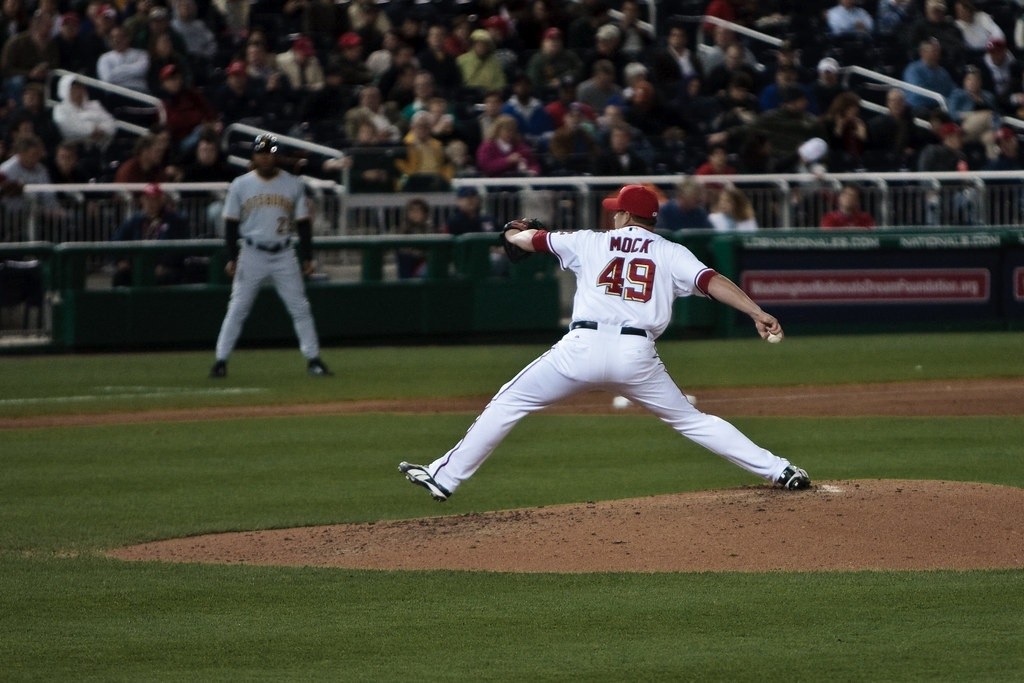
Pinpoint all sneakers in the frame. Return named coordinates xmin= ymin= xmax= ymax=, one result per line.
xmin=398 ymin=460 xmax=451 ymax=504
xmin=776 ymin=463 xmax=811 ymax=491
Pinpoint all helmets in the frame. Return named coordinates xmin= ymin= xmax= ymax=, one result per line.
xmin=250 ymin=132 xmax=279 ymax=154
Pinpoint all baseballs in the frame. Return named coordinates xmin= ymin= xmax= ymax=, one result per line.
xmin=766 ymin=331 xmax=783 ymax=344
xmin=612 ymin=396 xmax=631 ymax=410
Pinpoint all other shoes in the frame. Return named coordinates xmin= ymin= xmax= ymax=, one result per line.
xmin=307 ymin=359 xmax=327 ymax=375
xmin=210 ymin=360 xmax=226 ymax=378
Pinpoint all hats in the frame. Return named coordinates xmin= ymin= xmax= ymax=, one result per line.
xmin=937 ymin=122 xmax=967 ymax=137
xmin=293 ymin=35 xmax=318 ymax=57
xmin=60 ymin=15 xmax=81 ymax=29
xmin=471 ymin=30 xmax=491 ymax=44
xmin=335 ymin=31 xmax=365 ymax=48
xmin=995 ymin=128 xmax=1012 ymax=145
xmin=486 ymin=16 xmax=506 ymax=30
xmin=227 ymin=61 xmax=247 ymax=77
xmin=545 ymin=26 xmax=564 ymax=41
xmin=817 ymin=56 xmax=841 ymax=73
xmin=983 ymin=39 xmax=1007 ymax=54
xmin=797 ymin=136 xmax=829 ymax=163
xmin=601 ymin=184 xmax=659 ymax=220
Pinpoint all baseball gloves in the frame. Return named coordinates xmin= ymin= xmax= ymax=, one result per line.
xmin=501 ymin=217 xmax=548 ymax=263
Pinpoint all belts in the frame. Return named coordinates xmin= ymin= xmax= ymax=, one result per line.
xmin=245 ymin=237 xmax=290 ymax=254
xmin=569 ymin=319 xmax=648 ymax=339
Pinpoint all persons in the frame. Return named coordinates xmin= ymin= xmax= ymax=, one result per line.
xmin=0 ymin=0 xmax=1024 ymax=379
xmin=398 ymin=185 xmax=811 ymax=502
